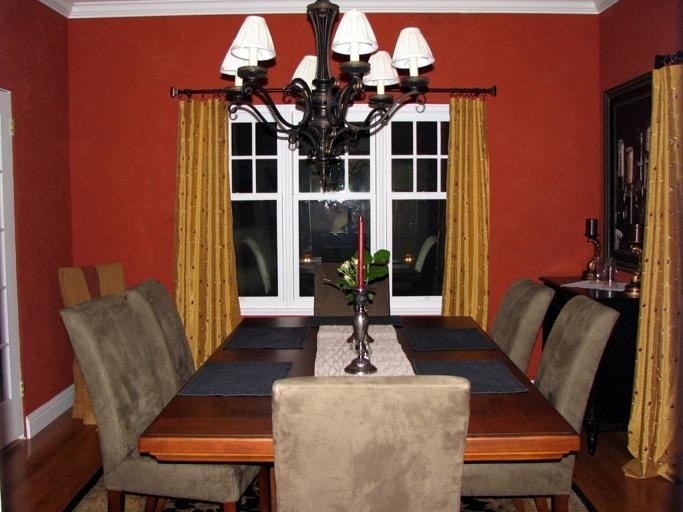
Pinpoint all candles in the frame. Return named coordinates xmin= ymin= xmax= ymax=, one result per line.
xmin=629 ymin=223 xmax=642 ymax=242
xmin=358 ymin=217 xmax=366 ymax=292
xmin=586 ymin=217 xmax=597 ymax=240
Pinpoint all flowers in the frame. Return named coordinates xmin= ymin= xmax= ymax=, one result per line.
xmin=333 ymin=249 xmax=389 ymax=303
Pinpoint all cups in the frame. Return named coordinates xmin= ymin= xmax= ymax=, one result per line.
xmin=605 ymin=267 xmax=615 ymax=288
xmin=596 ymin=257 xmax=604 ymax=284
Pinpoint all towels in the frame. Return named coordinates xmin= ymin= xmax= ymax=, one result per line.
xmin=309 ymin=315 xmax=404 ymax=327
xmin=222 ymin=325 xmax=309 ymax=349
xmin=404 ymin=326 xmax=497 ymax=351
xmin=411 ymin=358 xmax=529 ymax=395
xmin=177 ymin=360 xmax=294 ymax=397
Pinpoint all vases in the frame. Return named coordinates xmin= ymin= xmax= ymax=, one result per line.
xmin=348 ymin=289 xmax=374 ymax=344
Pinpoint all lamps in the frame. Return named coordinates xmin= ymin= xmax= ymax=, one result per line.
xmin=220 ymin=0 xmax=436 ymax=193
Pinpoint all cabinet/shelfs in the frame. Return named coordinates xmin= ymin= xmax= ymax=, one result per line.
xmin=540 ymin=274 xmax=642 ymax=453
xmin=603 ymin=70 xmax=653 ymax=279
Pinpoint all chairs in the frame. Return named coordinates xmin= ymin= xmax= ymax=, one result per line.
xmin=60 ymin=287 xmax=269 ymax=511
xmin=273 ymin=376 xmax=470 ymax=512
xmin=123 ymin=277 xmax=197 ymax=411
xmin=459 ymin=294 xmax=619 ymax=512
xmin=488 ymin=279 xmax=555 ymax=379
xmin=241 ymin=232 xmax=278 ymax=298
xmin=390 ymin=234 xmax=439 ymax=290
